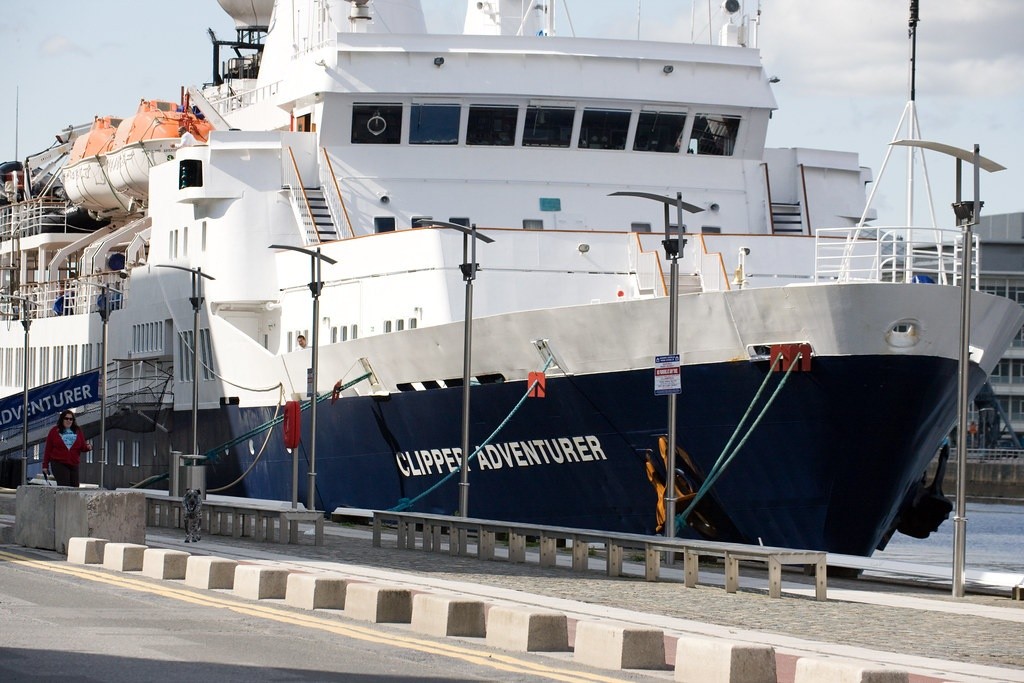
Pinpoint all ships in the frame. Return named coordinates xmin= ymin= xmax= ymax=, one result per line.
xmin=0 ymin=0 xmax=1024 ymax=583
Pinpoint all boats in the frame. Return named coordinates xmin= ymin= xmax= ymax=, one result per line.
xmin=896 ymin=211 xmax=1024 ymax=504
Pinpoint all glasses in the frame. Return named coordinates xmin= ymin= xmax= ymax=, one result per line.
xmin=64 ymin=416 xmax=74 ymax=421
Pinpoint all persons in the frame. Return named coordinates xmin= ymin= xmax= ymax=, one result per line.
xmin=293 ymin=334 xmax=312 ymax=352
xmin=43 ymin=409 xmax=93 ymax=488
